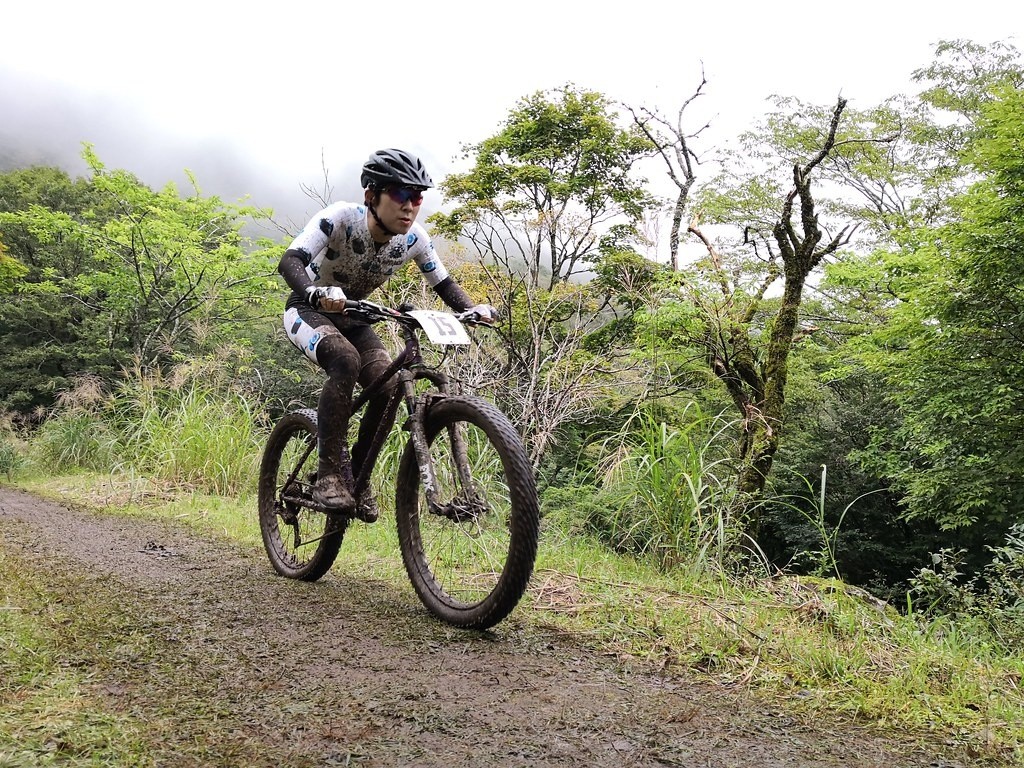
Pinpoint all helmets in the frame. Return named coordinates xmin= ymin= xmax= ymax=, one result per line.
xmin=361 ymin=149 xmax=432 ymax=187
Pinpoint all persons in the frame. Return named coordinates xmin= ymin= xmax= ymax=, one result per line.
xmin=277 ymin=147 xmax=499 ymax=524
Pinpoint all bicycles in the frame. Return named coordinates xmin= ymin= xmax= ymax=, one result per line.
xmin=258 ymin=299 xmax=540 ymax=632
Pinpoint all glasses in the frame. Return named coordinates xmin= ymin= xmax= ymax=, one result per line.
xmin=377 ymin=185 xmax=423 ymax=206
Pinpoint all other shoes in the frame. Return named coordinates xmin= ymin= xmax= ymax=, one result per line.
xmin=313 ymin=474 xmax=353 ymax=510
xmin=347 ymin=477 xmax=379 ymax=522
